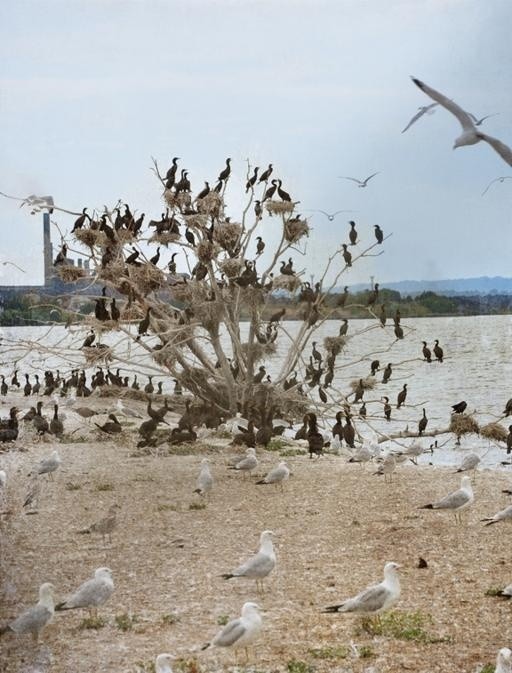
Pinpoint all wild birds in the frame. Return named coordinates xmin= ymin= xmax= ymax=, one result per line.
xmin=22 ymin=471 xmax=42 ymax=510
xmin=400 ymin=74 xmax=512 ymax=167
xmin=0 ymin=582 xmax=60 ymax=640
xmin=192 ymin=458 xmax=216 ymax=506
xmin=415 ymin=475 xmax=476 ymax=524
xmin=319 ymin=562 xmax=405 ymax=627
xmin=215 ymin=530 xmax=283 ymax=594
xmin=55 ymin=567 xmax=118 ymax=619
xmin=479 ymin=505 xmax=512 ymax=527
xmin=1 ymin=157 xmax=512 ymax=492
xmin=494 ymin=583 xmax=512 ymax=601
xmin=495 ymin=646 xmax=512 ymax=673
xmin=155 ymin=654 xmax=183 ymax=673
xmin=200 ymin=602 xmax=268 ymax=666
xmin=73 ymin=502 xmax=122 ymax=546
